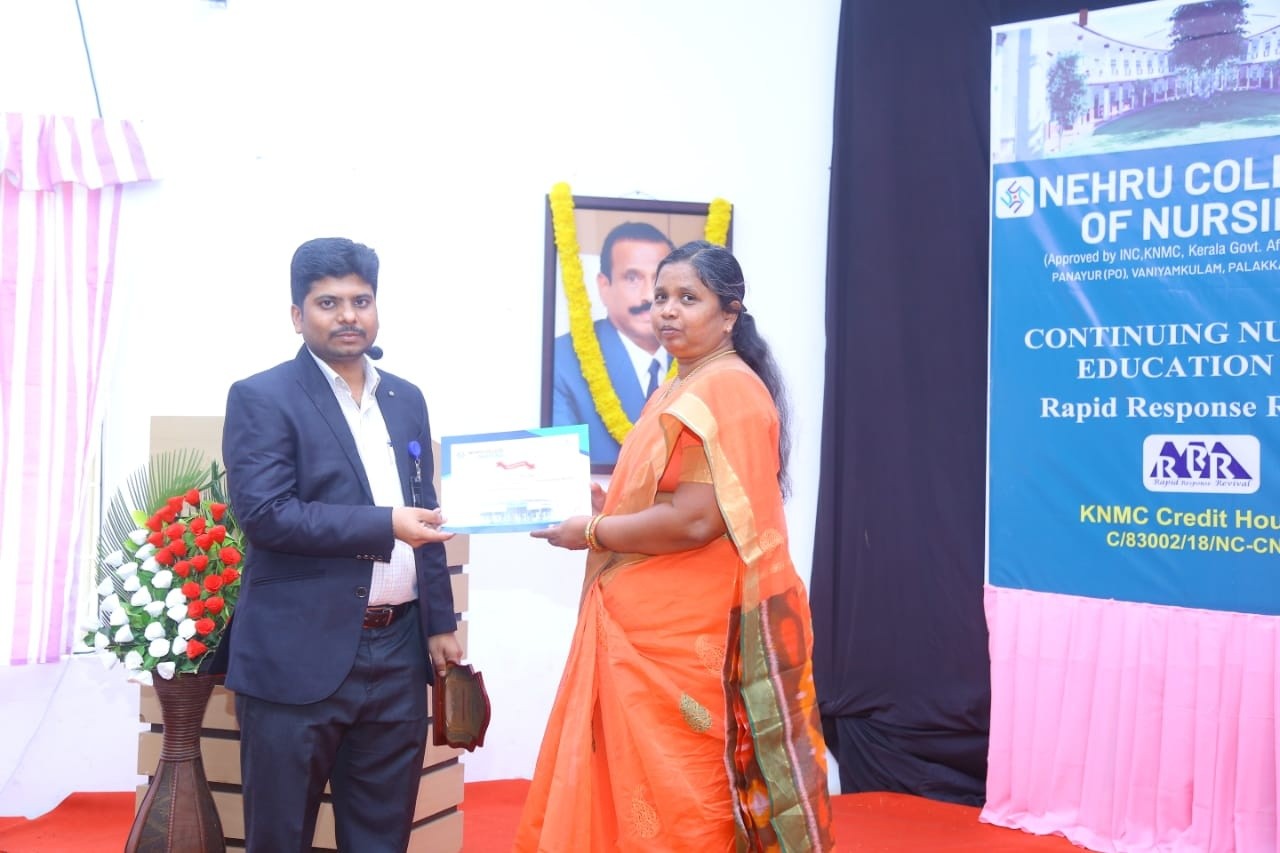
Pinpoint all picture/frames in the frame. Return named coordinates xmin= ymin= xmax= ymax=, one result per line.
xmin=540 ymin=196 xmax=736 ymax=476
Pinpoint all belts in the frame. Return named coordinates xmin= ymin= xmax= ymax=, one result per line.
xmin=362 ymin=602 xmax=412 ymax=629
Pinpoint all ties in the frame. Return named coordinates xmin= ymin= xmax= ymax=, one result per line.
xmin=648 ymin=358 xmax=660 ymax=398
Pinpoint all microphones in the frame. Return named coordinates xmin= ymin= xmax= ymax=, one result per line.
xmin=366 ymin=346 xmax=383 ymax=360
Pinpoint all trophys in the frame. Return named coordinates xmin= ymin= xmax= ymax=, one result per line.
xmin=434 ymin=658 xmax=491 ymax=753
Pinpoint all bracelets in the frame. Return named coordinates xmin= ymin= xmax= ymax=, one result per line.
xmin=584 ymin=512 xmax=613 ymax=553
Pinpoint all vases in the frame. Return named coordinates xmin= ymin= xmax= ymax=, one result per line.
xmin=124 ymin=664 xmax=226 ymax=853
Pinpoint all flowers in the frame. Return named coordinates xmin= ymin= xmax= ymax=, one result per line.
xmin=80 ymin=445 xmax=248 ymax=680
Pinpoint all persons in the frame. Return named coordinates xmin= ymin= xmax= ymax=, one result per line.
xmin=529 ymin=239 xmax=830 ymax=853
xmin=220 ymin=237 xmax=462 ymax=853
xmin=551 ymin=221 xmax=680 ymax=465
